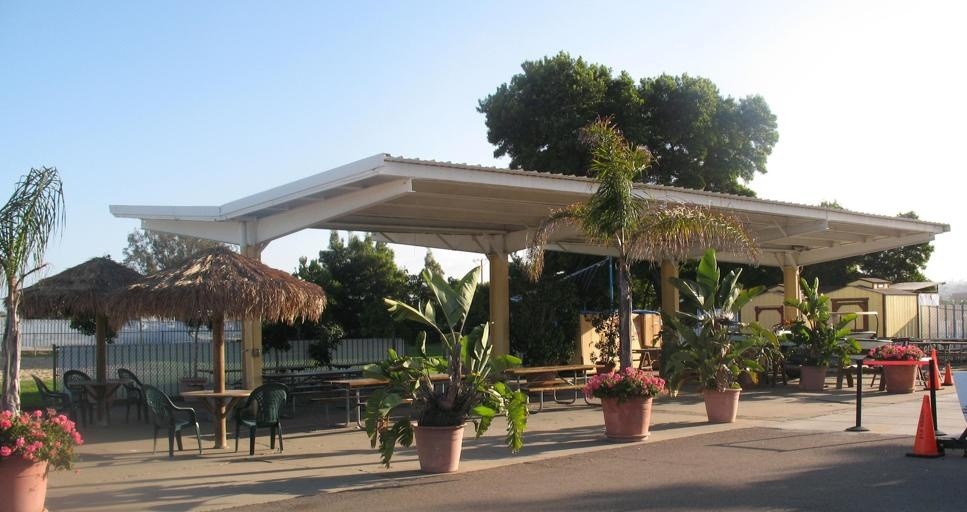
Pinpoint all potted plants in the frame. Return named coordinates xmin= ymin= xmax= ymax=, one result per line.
xmin=661 ymin=249 xmax=767 ymax=424
xmin=770 ymin=274 xmax=863 ymax=393
xmin=360 ymin=265 xmax=529 ymax=474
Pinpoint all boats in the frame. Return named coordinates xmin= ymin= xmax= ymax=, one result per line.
xmin=187 ymin=329 xmax=242 ymax=342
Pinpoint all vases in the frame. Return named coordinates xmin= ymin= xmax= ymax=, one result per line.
xmin=600 ymin=395 xmax=653 ymax=443
xmin=0 ymin=455 xmax=50 ymax=512
xmin=882 ymin=363 xmax=919 ymax=394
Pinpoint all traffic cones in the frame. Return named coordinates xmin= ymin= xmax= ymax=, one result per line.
xmin=903 ymin=394 xmax=943 ymax=460
xmin=943 ymin=360 xmax=955 ymax=386
xmin=924 ymin=348 xmax=943 ymax=391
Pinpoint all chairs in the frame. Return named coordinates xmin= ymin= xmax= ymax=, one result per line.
xmin=30 ymin=367 xmax=150 ymax=425
xmin=235 ymin=383 xmax=289 ymax=456
xmin=142 ymin=384 xmax=202 ymax=457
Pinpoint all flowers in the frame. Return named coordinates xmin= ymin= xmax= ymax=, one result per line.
xmin=0 ymin=406 xmax=83 ymax=477
xmin=581 ymin=366 xmax=668 ymax=402
xmin=868 ymin=344 xmax=929 ymax=361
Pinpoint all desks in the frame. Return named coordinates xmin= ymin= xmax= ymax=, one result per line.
xmin=502 ymin=363 xmax=605 ymax=414
xmin=261 ymin=369 xmax=454 ymax=432
xmin=179 ymin=389 xmax=254 ymax=450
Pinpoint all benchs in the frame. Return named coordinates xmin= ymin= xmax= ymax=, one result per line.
xmin=509 ymin=379 xmax=568 ymax=384
xmin=530 ymin=383 xmax=585 ymax=393
